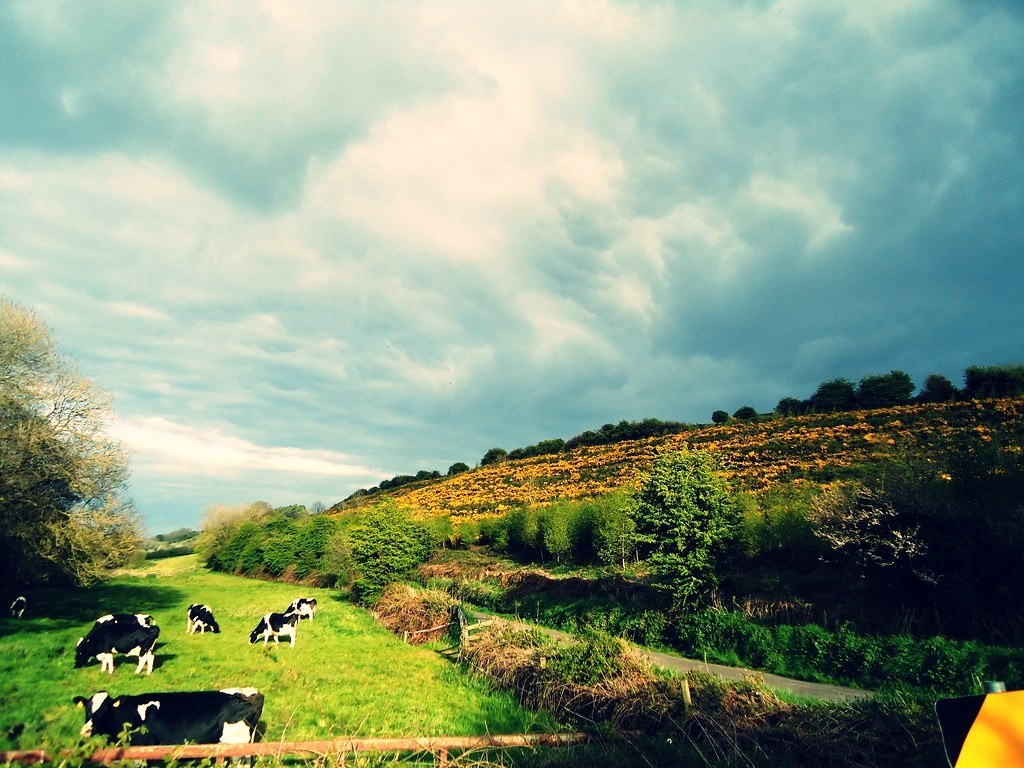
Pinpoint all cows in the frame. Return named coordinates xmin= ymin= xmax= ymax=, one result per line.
xmin=73 ymin=623 xmax=161 ymax=676
xmin=185 ymin=602 xmax=220 ymax=634
xmin=248 ymin=612 xmax=300 ymax=649
xmin=283 ymin=597 xmax=317 ymax=620
xmin=9 ymin=595 xmax=27 ymax=618
xmin=73 ymin=685 xmax=265 ymax=768
xmin=76 ymin=612 xmax=157 ymax=646
